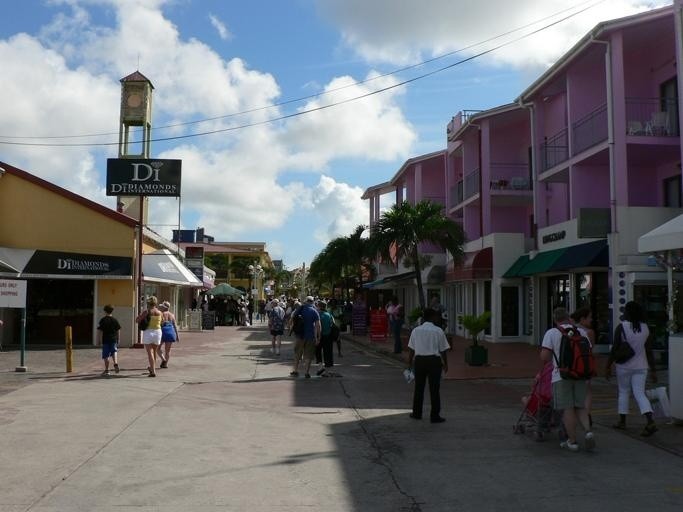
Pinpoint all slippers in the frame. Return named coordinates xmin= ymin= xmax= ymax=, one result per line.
xmin=316 ymin=368 xmax=325 ymax=375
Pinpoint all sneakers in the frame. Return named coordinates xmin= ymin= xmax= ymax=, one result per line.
xmin=148 ymin=373 xmax=156 ymax=377
xmin=289 ymin=371 xmax=298 ymax=376
xmin=114 ymin=363 xmax=120 ymax=373
xmin=161 ymin=359 xmax=166 ymax=368
xmin=640 ymin=423 xmax=658 ymax=437
xmin=160 ymin=363 xmax=168 ymax=368
xmin=270 ymin=348 xmax=275 ymax=354
xmin=613 ymin=421 xmax=625 ymax=430
xmin=276 ymin=348 xmax=280 ymax=355
xmin=584 ymin=432 xmax=595 ymax=449
xmin=101 ymin=370 xmax=110 ymax=376
xmin=147 ymin=366 xmax=152 ymax=373
xmin=305 ymin=373 xmax=311 ymax=378
xmin=559 ymin=439 xmax=579 ymax=451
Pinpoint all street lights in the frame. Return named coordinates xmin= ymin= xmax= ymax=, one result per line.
xmin=249 ymin=261 xmax=263 ymax=318
xmin=248 ymin=261 xmax=265 ymax=318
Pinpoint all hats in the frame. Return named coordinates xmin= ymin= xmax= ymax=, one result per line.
xmin=271 ymin=299 xmax=279 ymax=304
xmin=306 ymin=295 xmax=314 ymax=301
xmin=318 ymin=301 xmax=327 ymax=309
xmin=159 ymin=301 xmax=170 ymax=309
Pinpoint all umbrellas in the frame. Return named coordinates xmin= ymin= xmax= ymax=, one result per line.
xmin=199 ymin=283 xmax=244 ymax=295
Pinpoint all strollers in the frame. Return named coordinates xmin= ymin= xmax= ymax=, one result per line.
xmin=512 ymin=359 xmax=569 ymax=441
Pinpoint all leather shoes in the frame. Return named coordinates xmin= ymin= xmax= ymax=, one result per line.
xmin=431 ymin=416 xmax=446 ymax=424
xmin=409 ymin=412 xmax=422 ymax=420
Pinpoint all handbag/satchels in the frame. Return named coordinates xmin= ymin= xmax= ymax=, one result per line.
xmin=138 ymin=309 xmax=150 ymax=330
xmin=330 ymin=317 xmax=339 ymax=341
xmin=162 ymin=320 xmax=176 ymax=342
xmin=612 ymin=324 xmax=635 ymax=365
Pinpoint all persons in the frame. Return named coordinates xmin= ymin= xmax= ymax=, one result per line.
xmin=212 ymin=296 xmax=254 ymax=325
xmin=136 ymin=296 xmax=162 ymax=377
xmin=539 ymin=301 xmax=659 ymax=453
xmin=403 ymin=309 xmax=451 ymax=424
xmin=96 ymin=305 xmax=121 ymax=376
xmin=387 ymin=295 xmax=405 ymax=353
xmin=156 ymin=301 xmax=180 ymax=368
xmin=257 ymin=295 xmax=353 ymax=380
